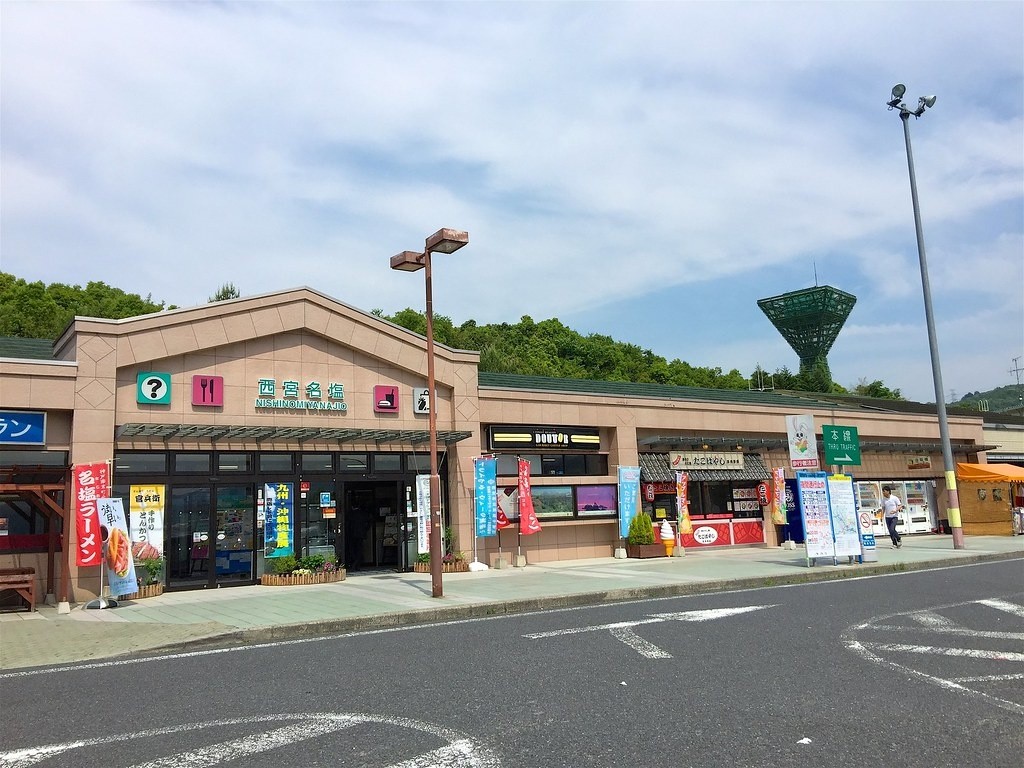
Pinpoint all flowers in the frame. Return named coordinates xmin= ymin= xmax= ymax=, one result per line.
xmin=292 ymin=568 xmax=312 ymax=575
xmin=315 ymin=552 xmax=341 ymax=574
xmin=417 ymin=527 xmax=471 ymax=564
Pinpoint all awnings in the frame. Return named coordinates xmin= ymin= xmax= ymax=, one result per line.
xmin=0 ymin=463 xmax=72 ymax=484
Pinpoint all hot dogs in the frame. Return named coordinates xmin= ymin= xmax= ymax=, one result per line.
xmin=107 ymin=528 xmax=130 ymax=578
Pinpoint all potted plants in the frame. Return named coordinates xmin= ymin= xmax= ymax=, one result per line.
xmin=626 ymin=511 xmax=667 ymax=558
xmin=141 ymin=553 xmax=166 ymax=585
xmin=267 ymin=552 xmax=298 ymax=577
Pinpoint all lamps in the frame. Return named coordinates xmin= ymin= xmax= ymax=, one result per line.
xmin=736 ymin=443 xmax=742 ymax=450
xmin=702 ymin=442 xmax=708 ymax=450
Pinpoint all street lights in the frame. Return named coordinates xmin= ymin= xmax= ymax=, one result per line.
xmin=888 ymin=83 xmax=964 ymax=551
xmin=390 ymin=227 xmax=468 ymax=601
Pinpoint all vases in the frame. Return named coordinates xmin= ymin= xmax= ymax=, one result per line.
xmin=456 ymin=559 xmax=462 ymax=562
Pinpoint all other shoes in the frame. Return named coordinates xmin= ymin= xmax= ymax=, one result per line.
xmin=897 ymin=538 xmax=902 ymax=547
xmin=891 ymin=544 xmax=897 ymax=549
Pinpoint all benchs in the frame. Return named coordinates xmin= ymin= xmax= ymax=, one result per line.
xmin=0 ymin=567 xmax=35 ymax=612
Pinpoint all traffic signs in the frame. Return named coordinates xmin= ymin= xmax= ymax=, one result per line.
xmin=823 ymin=425 xmax=862 ymax=467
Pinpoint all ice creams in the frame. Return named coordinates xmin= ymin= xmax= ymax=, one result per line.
xmin=660 ymin=519 xmax=675 ymax=555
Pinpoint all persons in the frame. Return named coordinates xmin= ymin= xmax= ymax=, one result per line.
xmin=880 ymin=485 xmax=903 ymax=549
xmin=710 ymin=495 xmax=719 ymax=513
xmin=592 ymin=502 xmax=599 ymax=510
